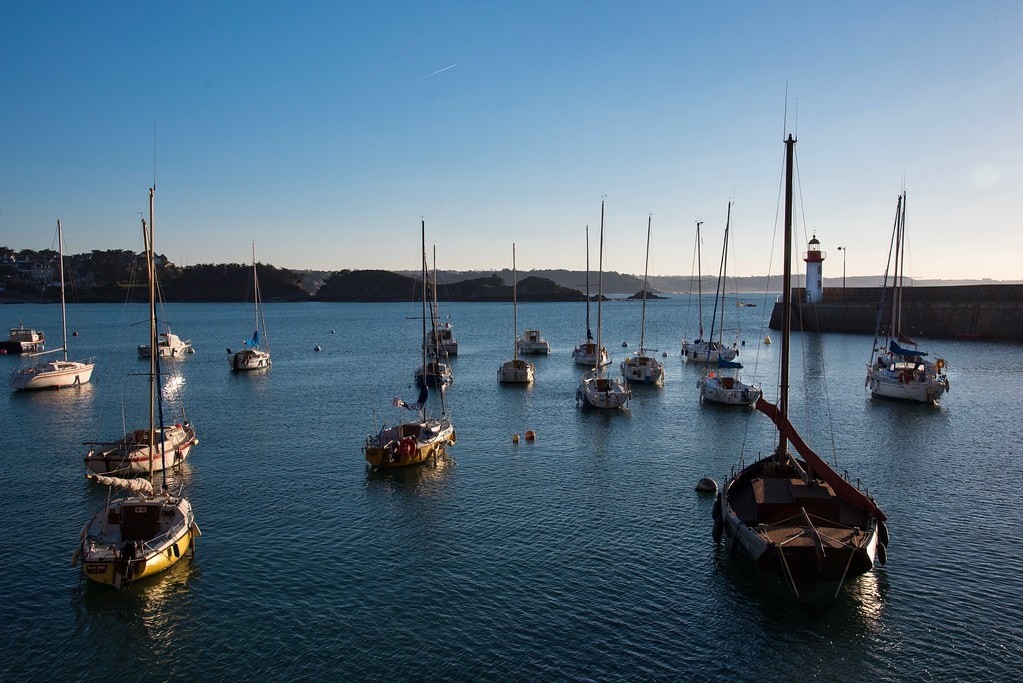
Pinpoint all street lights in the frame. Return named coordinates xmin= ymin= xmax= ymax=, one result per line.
xmin=837 ymin=245 xmax=847 ymax=305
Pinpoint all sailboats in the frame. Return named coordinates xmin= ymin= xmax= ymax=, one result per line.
xmin=136 ymin=269 xmax=195 ymax=358
xmin=497 ymin=243 xmax=535 ymax=383
xmin=6 ymin=219 xmax=98 ymax=392
xmin=411 ymin=243 xmax=455 ymax=393
xmin=576 ymin=195 xmax=633 ymax=410
xmin=619 ymin=212 xmax=663 ymax=386
xmin=358 ymin=219 xmax=456 ymax=469
xmin=712 ymin=75 xmax=891 ymax=600
xmin=572 ymin=224 xmax=609 ymax=368
xmin=696 ymin=197 xmax=761 ymax=405
xmin=864 ymin=168 xmax=949 ymax=403
xmin=225 ymin=239 xmax=273 ymax=371
xmin=681 ymin=220 xmax=742 ymax=364
xmin=80 ymin=212 xmax=200 ymax=478
xmin=77 ymin=183 xmax=196 ymax=592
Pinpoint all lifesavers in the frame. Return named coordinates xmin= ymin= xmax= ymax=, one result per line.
xmin=937 ymin=359 xmax=944 ymax=368
xmin=390 ymin=437 xmax=416 ymax=458
xmin=899 ymin=372 xmax=909 ymax=384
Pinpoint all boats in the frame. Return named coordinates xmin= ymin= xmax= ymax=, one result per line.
xmin=421 ymin=323 xmax=460 ymax=358
xmin=0 ymin=320 xmax=46 ymax=356
xmin=514 ymin=328 xmax=549 ymax=356
